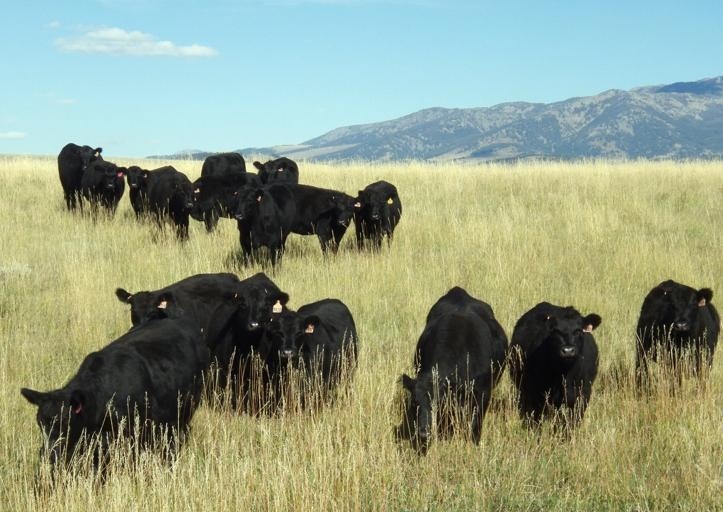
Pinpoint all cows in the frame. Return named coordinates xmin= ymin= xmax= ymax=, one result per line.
xmin=113 ymin=272 xmax=360 ymax=418
xmin=57 ymin=143 xmax=128 ymax=229
xmin=19 ymin=316 xmax=211 ymax=494
xmin=125 ymin=153 xmax=403 ymax=273
xmin=633 ymin=278 xmax=721 ymax=396
xmin=392 ymin=285 xmax=510 ymax=458
xmin=509 ymin=301 xmax=603 ymax=446
